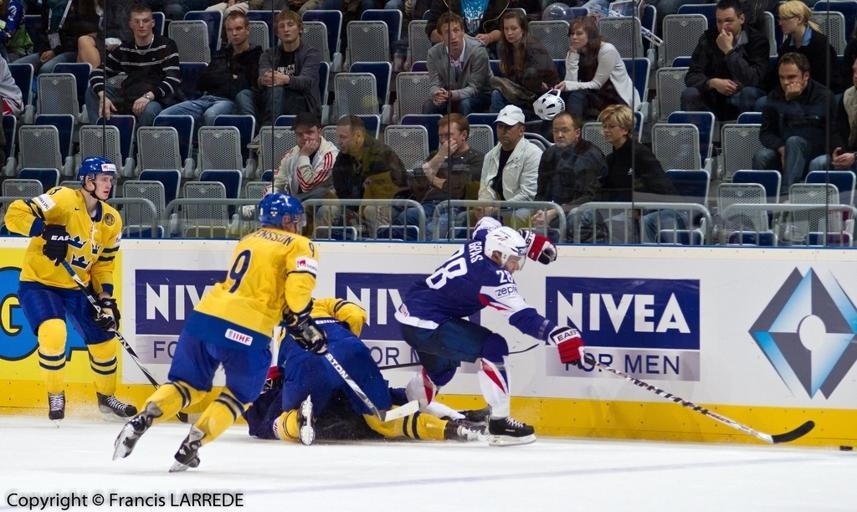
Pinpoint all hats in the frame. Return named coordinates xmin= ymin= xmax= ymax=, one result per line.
xmin=533 ymin=89 xmax=566 ymax=121
xmin=494 ymin=104 xmax=526 ymax=127
xmin=290 ymin=113 xmax=317 ymax=130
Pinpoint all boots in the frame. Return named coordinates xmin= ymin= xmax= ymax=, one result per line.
xmin=115 ymin=402 xmax=163 ymax=458
xmin=174 ymin=423 xmax=205 ymax=468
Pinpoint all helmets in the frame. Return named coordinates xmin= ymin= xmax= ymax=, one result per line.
xmin=257 ymin=193 xmax=307 ymax=227
xmin=79 ymin=156 xmax=117 ymax=185
xmin=484 ymin=227 xmax=529 ymax=271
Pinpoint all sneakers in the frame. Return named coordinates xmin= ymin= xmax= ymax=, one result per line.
xmin=48 ymin=391 xmax=66 ymax=419
xmin=96 ymin=392 xmax=138 ymax=417
xmin=488 ymin=415 xmax=534 ymax=436
xmin=441 ymin=407 xmax=489 ymax=441
xmin=299 ymin=399 xmax=317 ymax=445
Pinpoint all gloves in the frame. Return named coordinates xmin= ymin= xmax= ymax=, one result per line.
xmin=522 ymin=230 xmax=557 ymax=264
xmin=551 ymin=325 xmax=585 ymax=368
xmin=281 ymin=298 xmax=328 ymax=355
xmin=94 ymin=293 xmax=120 ymax=334
xmin=41 ymin=224 xmax=69 ymax=266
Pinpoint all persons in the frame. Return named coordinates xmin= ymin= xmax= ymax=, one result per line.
xmin=0 ymin=0 xmax=857 ymax=240
xmin=112 ymin=192 xmax=319 ymax=473
xmin=248 ymin=366 xmax=494 ymax=440
xmin=388 ymin=216 xmax=590 ymax=444
xmin=255 ymin=295 xmax=492 ymax=444
xmin=4 ymin=156 xmax=138 ymax=423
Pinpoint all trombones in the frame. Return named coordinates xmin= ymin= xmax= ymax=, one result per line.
xmin=60 ymin=256 xmax=203 ymax=423
